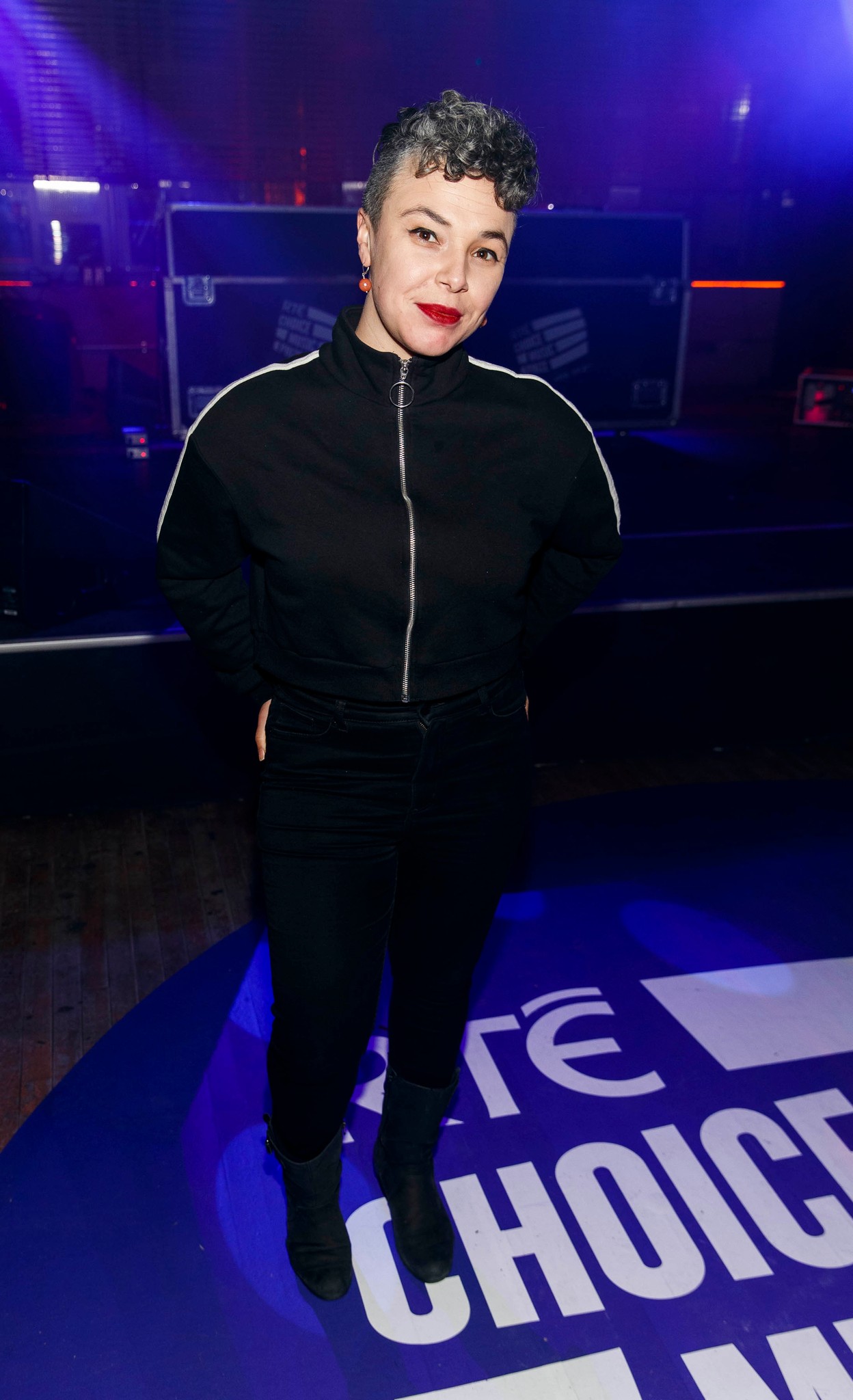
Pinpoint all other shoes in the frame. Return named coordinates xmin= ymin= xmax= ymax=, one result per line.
xmin=375 ymin=1158 xmax=455 ymax=1284
xmin=284 ymin=1163 xmax=356 ymax=1298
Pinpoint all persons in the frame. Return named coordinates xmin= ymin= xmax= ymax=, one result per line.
xmin=149 ymin=87 xmax=620 ymax=1303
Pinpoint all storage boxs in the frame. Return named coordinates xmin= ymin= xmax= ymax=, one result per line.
xmin=151 ymin=204 xmax=700 ymax=436
